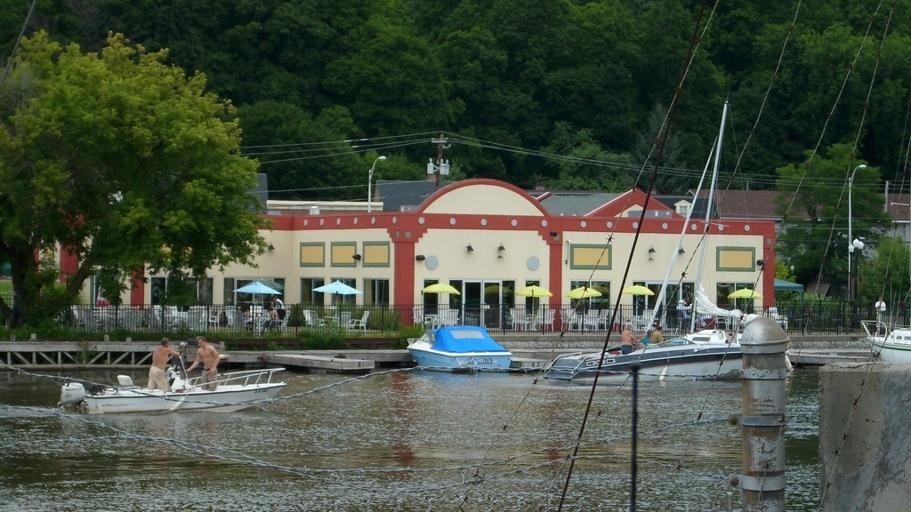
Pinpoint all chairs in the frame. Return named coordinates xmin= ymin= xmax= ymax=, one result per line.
xmin=301 ymin=309 xmax=370 ymax=337
xmin=509 ymin=306 xmax=788 ymax=332
xmin=70 ymin=304 xmax=291 ymax=335
xmin=412 ymin=308 xmax=459 ymax=331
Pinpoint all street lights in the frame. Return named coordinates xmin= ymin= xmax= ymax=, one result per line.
xmin=849 ymin=238 xmax=865 ymax=330
xmin=847 ymin=165 xmax=867 ymax=302
xmin=368 ymin=155 xmax=387 ymax=213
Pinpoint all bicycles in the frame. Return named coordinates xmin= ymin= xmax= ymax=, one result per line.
xmin=246 ymin=311 xmax=266 ymax=336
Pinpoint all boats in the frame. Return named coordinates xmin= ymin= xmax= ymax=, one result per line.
xmin=60 ymin=366 xmax=287 ymax=414
xmin=406 ymin=324 xmax=513 ymax=373
xmin=860 ymin=319 xmax=911 ymax=363
xmin=543 ymin=328 xmax=743 ymax=385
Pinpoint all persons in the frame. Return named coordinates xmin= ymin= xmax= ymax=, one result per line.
xmin=186 ymin=336 xmax=221 ymax=391
xmin=874 ymin=296 xmax=886 ymax=316
xmin=241 ymin=294 xmax=286 ymax=332
xmin=147 ymin=338 xmax=180 ymax=391
xmin=676 ymin=299 xmax=693 ymax=330
xmin=621 ymin=322 xmax=665 ymax=354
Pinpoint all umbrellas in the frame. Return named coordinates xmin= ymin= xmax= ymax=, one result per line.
xmin=422 ymin=282 xmax=461 ymax=314
xmin=485 ymin=285 xmax=513 ymax=296
xmin=516 ymin=284 xmax=553 ymax=316
xmin=584 ymin=285 xmax=608 ymax=294
xmin=312 ymin=278 xmax=363 ymax=317
xmin=565 ymin=286 xmax=602 ymax=322
xmin=241 ymin=278 xmax=284 ymax=306
xmin=620 ymin=284 xmax=655 ymax=316
xmin=232 ymin=280 xmax=282 ymax=306
xmin=727 ymin=288 xmax=763 ymax=313
